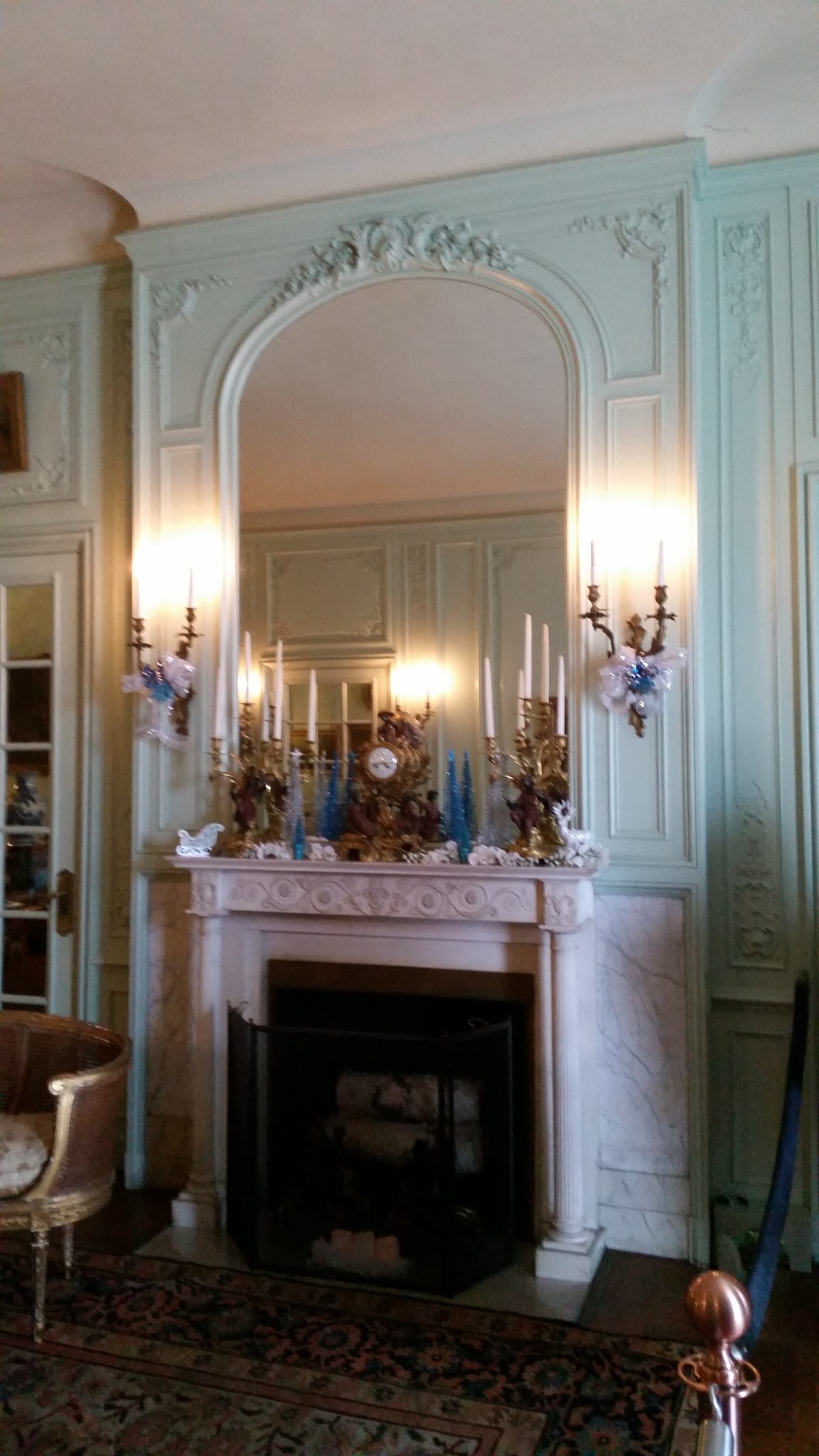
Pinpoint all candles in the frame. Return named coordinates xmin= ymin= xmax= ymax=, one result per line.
xmin=305 ymin=668 xmax=316 ymax=743
xmin=657 ymin=537 xmax=666 ymax=587
xmin=555 ymin=654 xmax=566 ymax=736
xmin=243 ymin=630 xmax=253 ymax=704
xmin=590 ymin=539 xmax=601 ymax=587
xmin=136 ymin=576 xmax=144 ymax=619
xmin=482 ymin=656 xmax=496 ymax=741
xmin=213 ymin=667 xmax=229 ymax=740
xmin=540 ymin=622 xmax=550 ymax=702
xmin=271 ymin=638 xmax=285 ymax=741
xmin=523 ymin=612 xmax=533 ymax=699
xmin=516 ymin=667 xmax=525 ymax=731
xmin=188 ymin=561 xmax=200 ymax=608
xmin=259 ymin=666 xmax=270 ymax=743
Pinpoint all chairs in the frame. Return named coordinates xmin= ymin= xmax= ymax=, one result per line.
xmin=0 ymin=1007 xmax=133 ymax=1346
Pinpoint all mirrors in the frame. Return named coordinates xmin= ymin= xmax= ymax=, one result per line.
xmin=238 ymin=279 xmax=569 ymax=852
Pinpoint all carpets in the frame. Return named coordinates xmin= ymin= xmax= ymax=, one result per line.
xmin=0 ymin=1226 xmax=710 ymax=1456
